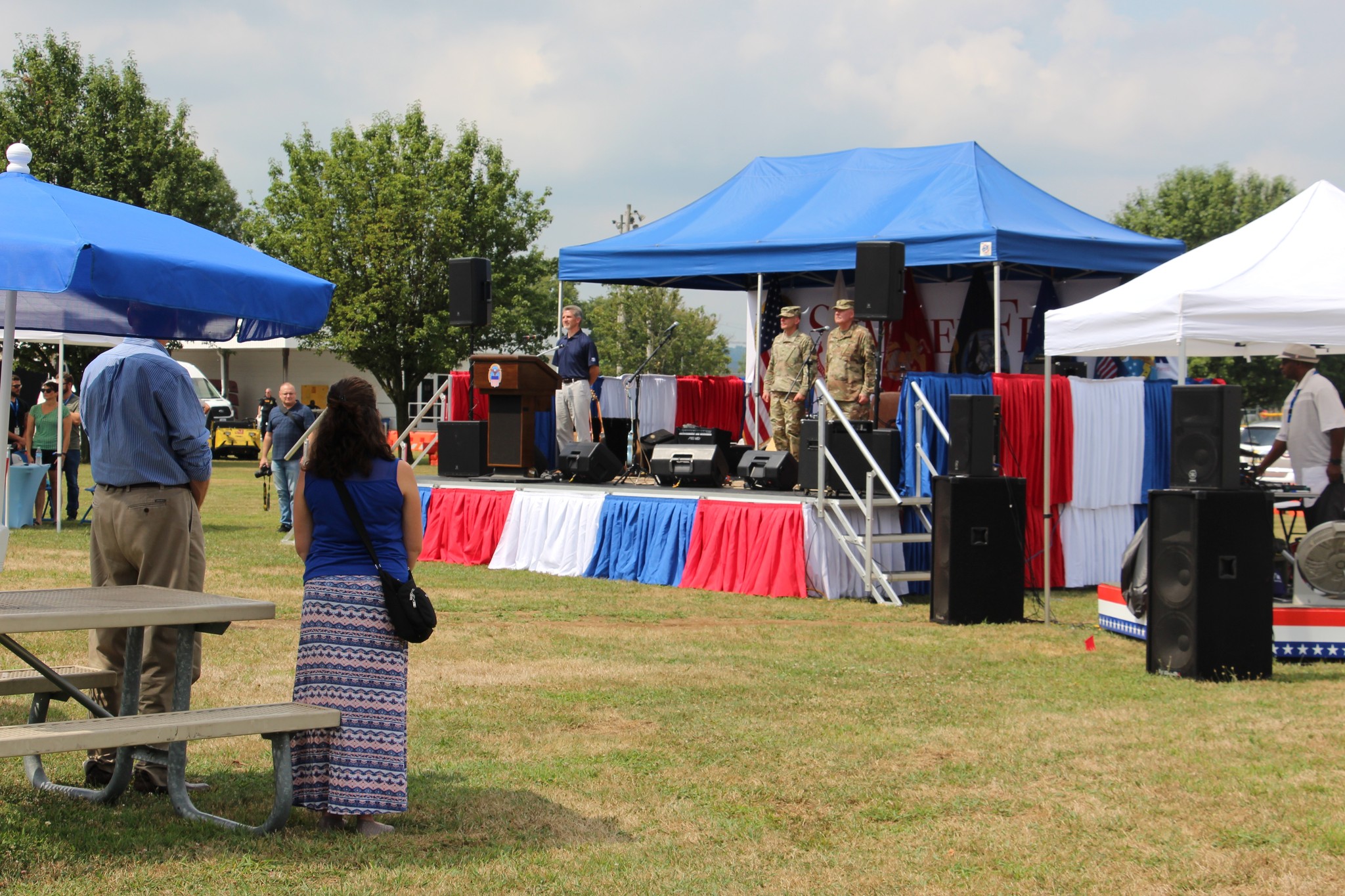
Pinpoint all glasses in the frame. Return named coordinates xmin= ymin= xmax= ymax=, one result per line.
xmin=40 ymin=388 xmax=52 ymax=394
xmin=13 ymin=384 xmax=22 ymax=388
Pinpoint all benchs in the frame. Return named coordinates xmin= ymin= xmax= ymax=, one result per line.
xmin=0 ymin=666 xmax=341 ymax=835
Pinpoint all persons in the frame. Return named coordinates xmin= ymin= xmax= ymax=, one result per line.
xmin=257 ymin=388 xmax=277 ymax=442
xmin=7 ymin=374 xmax=30 ymax=465
xmin=27 ymin=372 xmax=81 ymax=526
xmin=552 ymin=305 xmax=600 ymax=454
xmin=553 ymin=141 xmax=1187 ymax=482
xmin=259 ymin=382 xmax=316 ymax=533
xmin=306 ymin=400 xmax=321 ymax=420
xmin=823 ymin=299 xmax=876 ymax=421
xmin=1255 ymin=342 xmax=1345 ymax=533
xmin=761 ymin=305 xmax=817 ymax=464
xmin=290 ymin=375 xmax=423 ymax=837
xmin=78 ymin=300 xmax=213 ymax=794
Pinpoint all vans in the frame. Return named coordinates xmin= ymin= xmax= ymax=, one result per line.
xmin=174 ymin=360 xmax=236 ymax=425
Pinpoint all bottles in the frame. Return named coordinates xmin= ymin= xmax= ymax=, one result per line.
xmin=35 ymin=446 xmax=42 ymax=465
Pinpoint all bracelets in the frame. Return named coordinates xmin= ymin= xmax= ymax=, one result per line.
xmin=62 ymin=452 xmax=66 ymax=456
xmin=1330 ymin=459 xmax=1341 ymax=464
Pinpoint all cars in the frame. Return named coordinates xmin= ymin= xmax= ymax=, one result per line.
xmin=1239 ymin=420 xmax=1297 ymax=484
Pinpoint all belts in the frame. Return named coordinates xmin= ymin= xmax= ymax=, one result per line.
xmin=563 ymin=377 xmax=585 ymax=383
xmin=98 ymin=482 xmax=190 ymax=488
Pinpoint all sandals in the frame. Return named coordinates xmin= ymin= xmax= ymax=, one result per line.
xmin=32 ymin=521 xmax=40 ymax=526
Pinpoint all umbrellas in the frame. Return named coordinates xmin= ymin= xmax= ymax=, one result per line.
xmin=0 ymin=142 xmax=336 ymax=574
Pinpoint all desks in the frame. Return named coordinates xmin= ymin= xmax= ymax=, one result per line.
xmin=1272 ymin=492 xmax=1320 ymax=586
xmin=2 ymin=464 xmax=51 ymax=529
xmin=0 ymin=585 xmax=293 ymax=835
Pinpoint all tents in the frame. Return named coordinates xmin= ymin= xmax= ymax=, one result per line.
xmin=1043 ymin=178 xmax=1345 ymax=629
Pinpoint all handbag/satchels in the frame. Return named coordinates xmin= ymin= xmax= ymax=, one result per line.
xmin=382 ymin=570 xmax=438 ymax=643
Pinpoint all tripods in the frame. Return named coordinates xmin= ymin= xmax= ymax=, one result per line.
xmin=613 ymin=329 xmax=674 ymax=485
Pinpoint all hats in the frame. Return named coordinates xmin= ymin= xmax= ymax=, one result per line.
xmin=1276 ymin=343 xmax=1321 ymax=364
xmin=831 ymin=300 xmax=854 ymax=309
xmin=777 ymin=305 xmax=801 ymax=318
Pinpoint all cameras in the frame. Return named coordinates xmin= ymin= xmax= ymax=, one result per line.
xmin=254 ymin=464 xmax=272 ymax=479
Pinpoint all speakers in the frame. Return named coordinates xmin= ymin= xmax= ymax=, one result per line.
xmin=1147 ymin=488 xmax=1273 ymax=681
xmin=437 ymin=420 xmax=493 ymax=477
xmin=930 ymin=475 xmax=1026 ymax=626
xmin=449 ymin=257 xmax=491 ymax=329
xmin=1170 ymin=384 xmax=1241 ymax=491
xmin=558 ymin=418 xmax=640 ymax=484
xmin=947 ymin=394 xmax=1001 ymax=477
xmin=1024 ymin=362 xmax=1087 ymax=379
xmin=855 ymin=241 xmax=907 ymax=321
xmin=649 ymin=419 xmax=902 ymax=496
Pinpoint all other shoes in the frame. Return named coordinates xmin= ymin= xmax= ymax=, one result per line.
xmin=276 ymin=523 xmax=291 ymax=533
xmin=357 ymin=820 xmax=393 ymax=834
xmin=316 ymin=815 xmax=348 ymax=831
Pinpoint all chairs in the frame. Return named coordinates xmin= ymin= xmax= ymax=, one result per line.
xmin=80 ymin=484 xmax=96 ymax=524
xmin=11 ymin=450 xmax=55 ymax=521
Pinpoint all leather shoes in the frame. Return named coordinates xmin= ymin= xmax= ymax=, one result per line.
xmin=86 ymin=767 xmax=112 ymax=784
xmin=134 ymin=772 xmax=211 ymax=794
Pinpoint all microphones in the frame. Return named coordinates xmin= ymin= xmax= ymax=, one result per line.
xmin=538 ymin=344 xmax=565 ymax=359
xmin=523 ymin=334 xmax=542 ymax=340
xmin=664 ymin=321 xmax=679 ymax=333
xmin=810 ymin=325 xmax=829 ymax=332
